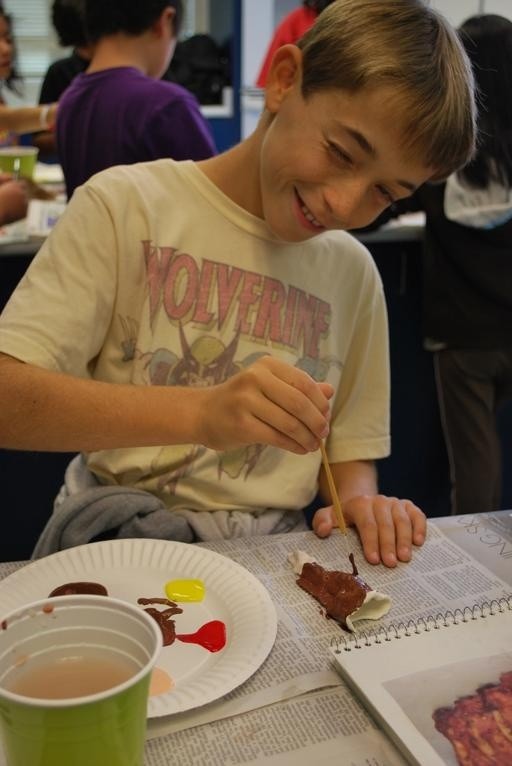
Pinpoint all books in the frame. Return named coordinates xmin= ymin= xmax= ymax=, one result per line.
xmin=328 ymin=592 xmax=512 ymax=766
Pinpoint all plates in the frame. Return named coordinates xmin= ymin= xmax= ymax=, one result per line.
xmin=0 ymin=537 xmax=279 ymax=723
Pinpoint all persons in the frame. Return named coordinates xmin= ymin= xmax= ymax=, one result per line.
xmin=1 ymin=4 xmax=67 ymax=146
xmin=1 ymin=0 xmax=485 ymax=569
xmin=35 ymin=33 xmax=95 ymax=195
xmin=55 ymin=0 xmax=216 ymax=199
xmin=258 ymin=0 xmax=336 ymax=90
xmin=1 ymin=175 xmax=30 ymax=226
xmin=344 ymin=14 xmax=511 ymax=520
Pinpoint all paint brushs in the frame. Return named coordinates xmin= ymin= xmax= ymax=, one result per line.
xmin=319 ymin=438 xmax=358 ymax=576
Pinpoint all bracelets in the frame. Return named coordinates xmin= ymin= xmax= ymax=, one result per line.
xmin=38 ymin=103 xmax=52 ymax=131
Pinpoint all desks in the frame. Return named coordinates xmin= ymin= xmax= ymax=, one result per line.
xmin=1 ymin=159 xmax=508 ymax=415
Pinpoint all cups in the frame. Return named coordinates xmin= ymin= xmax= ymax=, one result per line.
xmin=0 ymin=591 xmax=165 ymax=766
xmin=0 ymin=145 xmax=40 ymax=183
